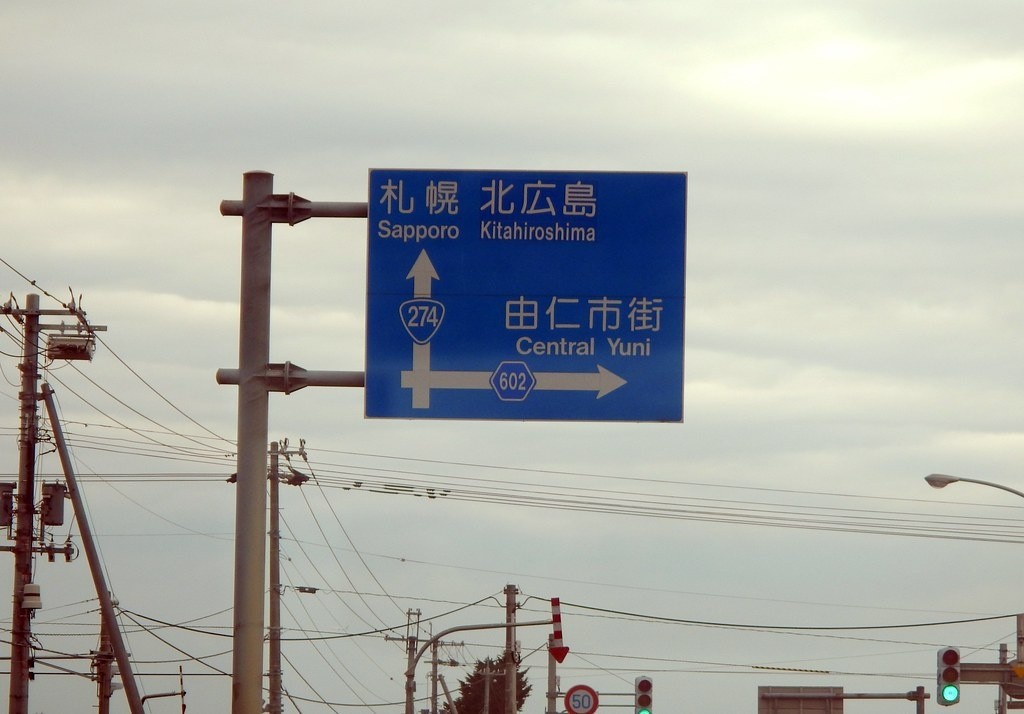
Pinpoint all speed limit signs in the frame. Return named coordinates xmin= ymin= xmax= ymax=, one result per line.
xmin=564 ymin=685 xmax=599 ymax=714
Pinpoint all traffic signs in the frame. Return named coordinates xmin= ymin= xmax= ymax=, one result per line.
xmin=367 ymin=170 xmax=685 ymax=422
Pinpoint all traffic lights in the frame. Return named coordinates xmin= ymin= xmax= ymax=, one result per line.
xmin=635 ymin=677 xmax=652 ymax=714
xmin=109 ymin=664 xmax=123 ymax=699
xmin=936 ymin=645 xmax=960 ymax=704
